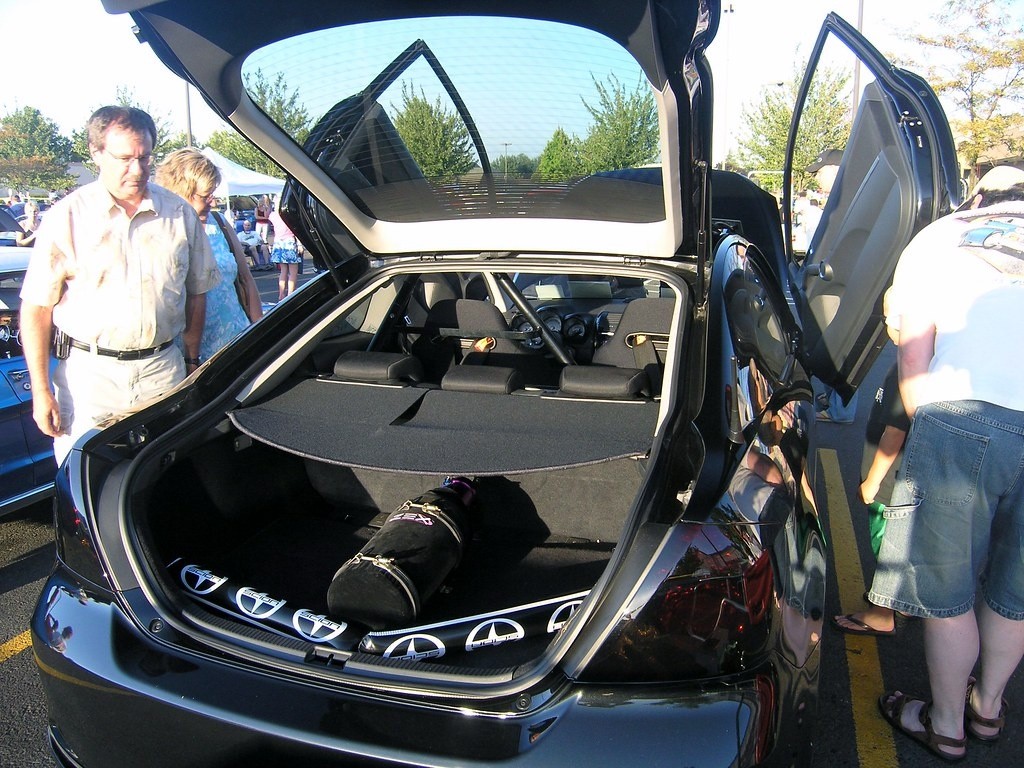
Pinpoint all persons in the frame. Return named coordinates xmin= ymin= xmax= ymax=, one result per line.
xmin=832 ymin=286 xmax=912 ymax=635
xmin=237 ymin=220 xmax=262 ymax=265
xmin=802 ymin=199 xmax=823 ymax=250
xmin=14 ymin=195 xmax=21 ymax=203
xmin=815 ymin=383 xmax=858 ymax=424
xmin=20 ymin=106 xmax=221 ymax=469
xmin=268 ymin=192 xmax=303 ymax=301
xmin=2 ymin=200 xmax=12 ymax=207
xmin=16 ymin=201 xmax=41 ymax=248
xmin=255 ymin=195 xmax=271 ymax=244
xmin=65 ymin=186 xmax=73 ymax=195
xmin=49 ymin=189 xmax=58 ymax=206
xmin=879 ymin=166 xmax=1024 ymax=764
xmin=805 ymin=149 xmax=843 ymax=192
xmin=155 ymin=148 xmax=263 ymax=366
xmin=794 ymin=192 xmax=810 ymax=225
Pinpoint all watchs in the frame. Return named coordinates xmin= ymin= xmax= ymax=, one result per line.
xmin=185 ymin=356 xmax=201 ymax=366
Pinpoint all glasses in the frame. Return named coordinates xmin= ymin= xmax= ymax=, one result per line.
xmin=193 ymin=192 xmax=214 ymax=204
xmin=104 ymin=148 xmax=154 ymax=167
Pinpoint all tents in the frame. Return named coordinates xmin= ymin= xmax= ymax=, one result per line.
xmin=201 ymin=147 xmax=286 ymax=211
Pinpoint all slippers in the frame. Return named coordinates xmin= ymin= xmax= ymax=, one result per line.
xmin=831 ymin=614 xmax=896 ymax=636
xmin=863 ymin=591 xmax=917 ymax=622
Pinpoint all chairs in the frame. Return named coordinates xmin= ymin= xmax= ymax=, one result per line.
xmin=411 ymin=299 xmax=538 ymax=377
xmin=236 ymin=220 xmax=274 ymax=269
xmin=590 ymin=297 xmax=677 ymax=372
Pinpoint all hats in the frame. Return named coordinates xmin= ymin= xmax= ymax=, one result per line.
xmin=805 ymin=149 xmax=843 ymax=173
xmin=952 ymin=165 xmax=1024 ymax=214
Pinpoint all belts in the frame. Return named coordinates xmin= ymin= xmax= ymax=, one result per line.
xmin=69 ymin=338 xmax=173 ymax=360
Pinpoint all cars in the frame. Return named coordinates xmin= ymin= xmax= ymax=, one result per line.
xmin=29 ymin=0 xmax=1024 ymax=768
xmin=1 ymin=245 xmax=90 ymax=522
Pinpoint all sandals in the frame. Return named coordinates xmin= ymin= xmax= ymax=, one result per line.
xmin=878 ymin=690 xmax=968 ymax=762
xmin=965 ymin=676 xmax=1009 ymax=745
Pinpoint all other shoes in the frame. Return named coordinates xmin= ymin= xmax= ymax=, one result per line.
xmin=265 ymin=264 xmax=274 ymax=270
xmin=258 ymin=265 xmax=264 ymax=271
